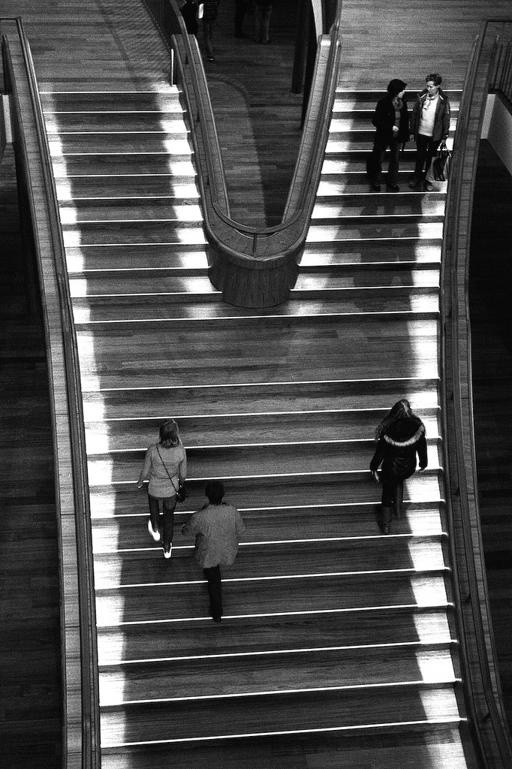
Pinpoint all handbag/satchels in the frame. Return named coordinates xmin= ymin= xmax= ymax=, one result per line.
xmin=174 ymin=482 xmax=188 ymax=503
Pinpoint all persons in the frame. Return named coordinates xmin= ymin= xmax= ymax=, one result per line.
xmin=368 ymin=398 xmax=428 ymax=534
xmin=181 ymin=478 xmax=246 ymax=622
xmin=136 ymin=419 xmax=188 ymax=559
xmin=367 ymin=77 xmax=410 ymax=193
xmin=407 ymin=72 xmax=451 ymax=189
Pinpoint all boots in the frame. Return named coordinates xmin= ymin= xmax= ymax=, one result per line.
xmin=392 ymin=486 xmax=404 ymax=520
xmin=377 ymin=505 xmax=393 ymax=536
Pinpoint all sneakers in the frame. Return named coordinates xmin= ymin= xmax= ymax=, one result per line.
xmin=145 ymin=518 xmax=161 ymax=543
xmin=161 ymin=541 xmax=174 ymax=561
